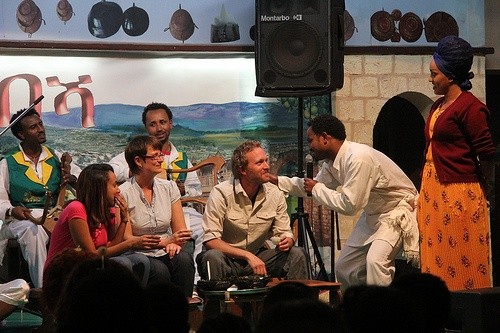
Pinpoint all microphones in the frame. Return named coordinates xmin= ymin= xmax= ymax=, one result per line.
xmin=306 ymin=154 xmax=313 ymax=196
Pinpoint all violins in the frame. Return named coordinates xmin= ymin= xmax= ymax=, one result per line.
xmin=38 ymin=152 xmax=73 ymax=234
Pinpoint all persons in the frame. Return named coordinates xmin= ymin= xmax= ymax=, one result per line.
xmin=196 ymin=139 xmax=313 ymax=320
xmin=0 ymin=108 xmax=83 ymax=288
xmin=268 ymin=113 xmax=420 ymax=298
xmin=116 ymin=133 xmax=195 ymax=298
xmin=42 ymin=248 xmax=454 ymax=333
xmin=108 ymin=102 xmax=204 ymax=306
xmin=415 ymin=35 xmax=500 ymax=291
xmin=0 ymin=279 xmax=32 ymax=322
xmin=41 ymin=163 xmax=161 ymax=290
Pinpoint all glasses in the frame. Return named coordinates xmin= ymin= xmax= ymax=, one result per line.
xmin=142 ymin=153 xmax=164 ymax=159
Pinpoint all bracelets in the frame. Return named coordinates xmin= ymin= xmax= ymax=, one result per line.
xmin=9 ymin=207 xmax=15 ymax=216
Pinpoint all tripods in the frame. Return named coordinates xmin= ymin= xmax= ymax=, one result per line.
xmin=289 ymin=97 xmax=330 ymax=283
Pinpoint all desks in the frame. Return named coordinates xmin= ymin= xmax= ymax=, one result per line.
xmin=196 ymin=279 xmax=343 ymax=327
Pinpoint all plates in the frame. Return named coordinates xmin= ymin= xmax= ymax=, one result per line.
xmin=197 ymin=280 xmax=231 ymax=290
xmin=234 ymin=275 xmax=272 ymax=288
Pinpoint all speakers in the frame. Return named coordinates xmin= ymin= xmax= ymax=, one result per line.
xmin=254 ymin=0 xmax=345 ymax=97
